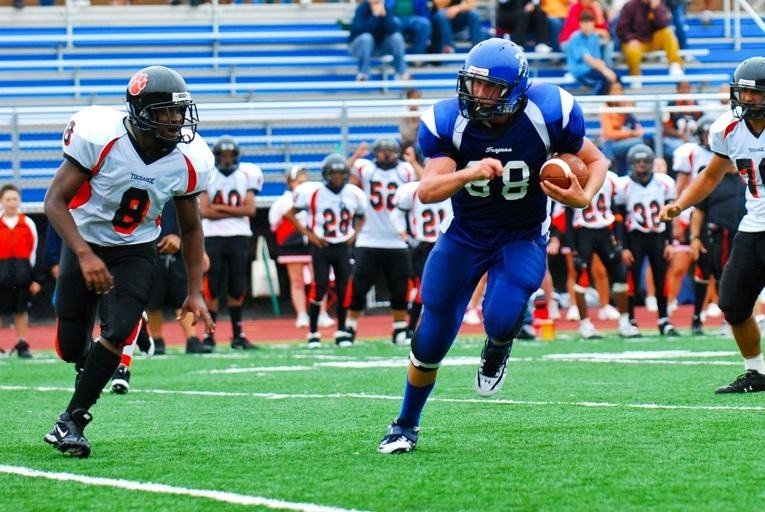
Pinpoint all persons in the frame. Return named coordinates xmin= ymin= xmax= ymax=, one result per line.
xmin=268 ymin=138 xmax=424 ymax=348
xmin=377 ymin=37 xmax=611 ymax=453
xmin=349 ymin=0 xmax=689 ymax=96
xmin=40 ymin=66 xmax=216 ymax=457
xmin=197 ymin=134 xmax=265 ymax=350
xmin=1 ymin=186 xmax=40 ymax=359
xmin=599 ymin=82 xmax=763 ymax=336
xmin=657 ymin=56 xmax=764 ymax=394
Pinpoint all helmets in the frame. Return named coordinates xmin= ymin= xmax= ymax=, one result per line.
xmin=696 ymin=119 xmax=715 ymax=134
xmin=322 ymin=153 xmax=350 ymax=183
xmin=213 ymin=136 xmax=239 ymax=171
xmin=372 ymin=136 xmax=401 ymax=164
xmin=126 ymin=64 xmax=199 ymax=145
xmin=627 ymin=144 xmax=655 ymax=184
xmin=456 ymin=38 xmax=533 ymax=120
xmin=729 ymin=57 xmax=765 ymax=123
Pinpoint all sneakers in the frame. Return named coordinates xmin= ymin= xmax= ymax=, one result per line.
xmin=44 ymin=409 xmax=93 ymax=459
xmin=669 ymin=61 xmax=684 ymax=75
xmin=717 ymin=369 xmax=765 ymax=394
xmin=535 ymin=41 xmax=551 ymax=52
xmin=17 ymin=340 xmax=31 ymax=356
xmin=392 ymin=328 xmax=415 ymax=345
xmin=296 ymin=313 xmax=357 ymax=349
xmin=376 ymin=417 xmax=420 ymax=454
xmin=112 ymin=365 xmax=131 ymax=393
xmin=463 ymin=311 xmax=481 ymax=325
xmin=519 ymin=284 xmax=722 ymax=339
xmin=473 ymin=336 xmax=514 ymax=397
xmin=230 ymin=338 xmax=257 ymax=351
xmin=185 ymin=333 xmax=215 ymax=354
xmin=137 ymin=310 xmax=164 ymax=357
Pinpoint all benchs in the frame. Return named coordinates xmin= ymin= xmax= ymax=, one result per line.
xmin=0 ymin=31 xmax=732 ymax=201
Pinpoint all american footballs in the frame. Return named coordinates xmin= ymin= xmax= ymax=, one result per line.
xmin=539 ymin=154 xmax=589 ymax=190
